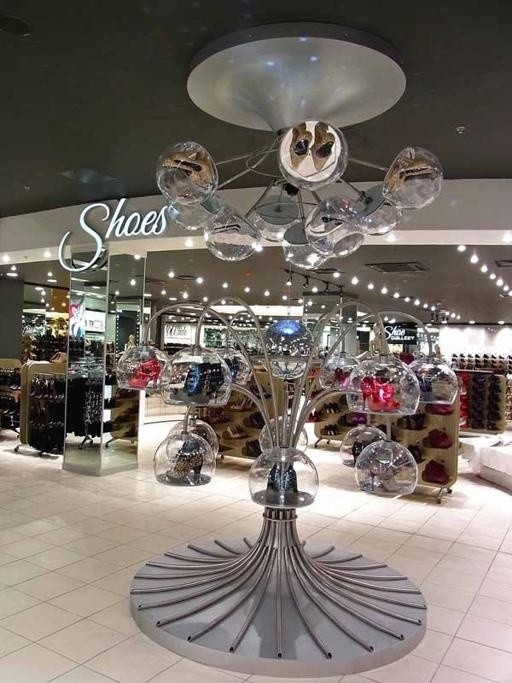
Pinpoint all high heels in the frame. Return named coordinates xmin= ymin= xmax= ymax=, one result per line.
xmin=129 ymin=359 xmax=224 ymax=483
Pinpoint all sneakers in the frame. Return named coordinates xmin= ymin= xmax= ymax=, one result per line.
xmin=451 ymin=354 xmax=512 ymax=429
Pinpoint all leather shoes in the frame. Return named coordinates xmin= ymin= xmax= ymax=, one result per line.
xmin=316 ymin=353 xmax=456 ymax=492
xmin=210 ymin=382 xmax=271 ymax=457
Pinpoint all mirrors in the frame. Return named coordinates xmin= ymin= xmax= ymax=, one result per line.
xmin=62 ymin=253 xmax=146 ymax=476
